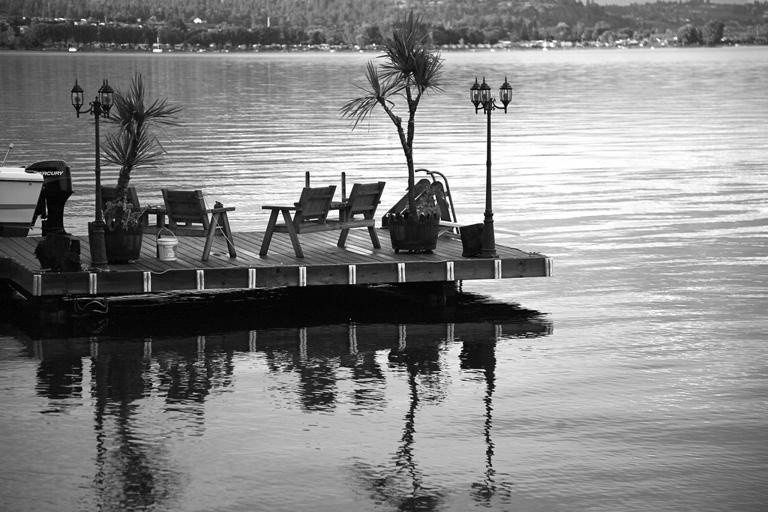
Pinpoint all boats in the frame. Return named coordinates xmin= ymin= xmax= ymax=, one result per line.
xmin=0 ymin=137 xmax=43 ymax=236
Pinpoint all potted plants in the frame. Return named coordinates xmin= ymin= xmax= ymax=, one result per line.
xmin=97 ymin=70 xmax=185 ymax=266
xmin=337 ymin=7 xmax=446 ymax=254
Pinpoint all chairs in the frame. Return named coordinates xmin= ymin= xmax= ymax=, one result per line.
xmin=258 ymin=181 xmax=385 ymax=258
xmin=95 ymin=185 xmax=237 ymax=261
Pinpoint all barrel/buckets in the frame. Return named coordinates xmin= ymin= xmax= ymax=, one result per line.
xmin=459 ymin=222 xmax=483 ymax=258
xmin=87 ymin=219 xmax=108 ymax=266
xmin=155 ymin=229 xmax=178 ymax=263
xmin=387 ymin=212 xmax=442 ymax=251
xmin=109 ymin=230 xmax=141 ymax=263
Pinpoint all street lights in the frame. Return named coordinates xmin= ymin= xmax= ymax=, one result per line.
xmin=70 ymin=73 xmax=115 ymax=272
xmin=469 ymin=73 xmax=512 ymax=262
